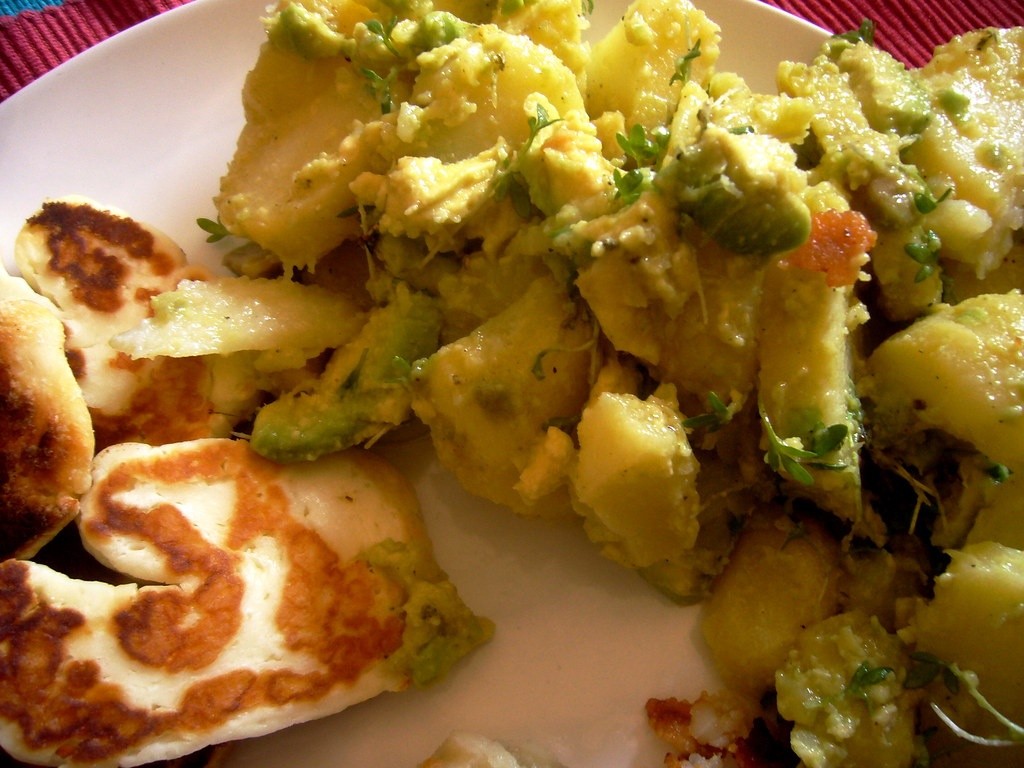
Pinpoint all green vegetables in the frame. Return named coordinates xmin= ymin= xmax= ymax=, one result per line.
xmin=201 ymin=0 xmax=1024 ymax=754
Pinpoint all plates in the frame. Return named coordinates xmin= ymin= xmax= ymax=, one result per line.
xmin=0 ymin=2 xmax=1023 ymax=768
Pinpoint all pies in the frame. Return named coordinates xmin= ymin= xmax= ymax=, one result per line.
xmin=0 ymin=197 xmax=467 ymax=768
